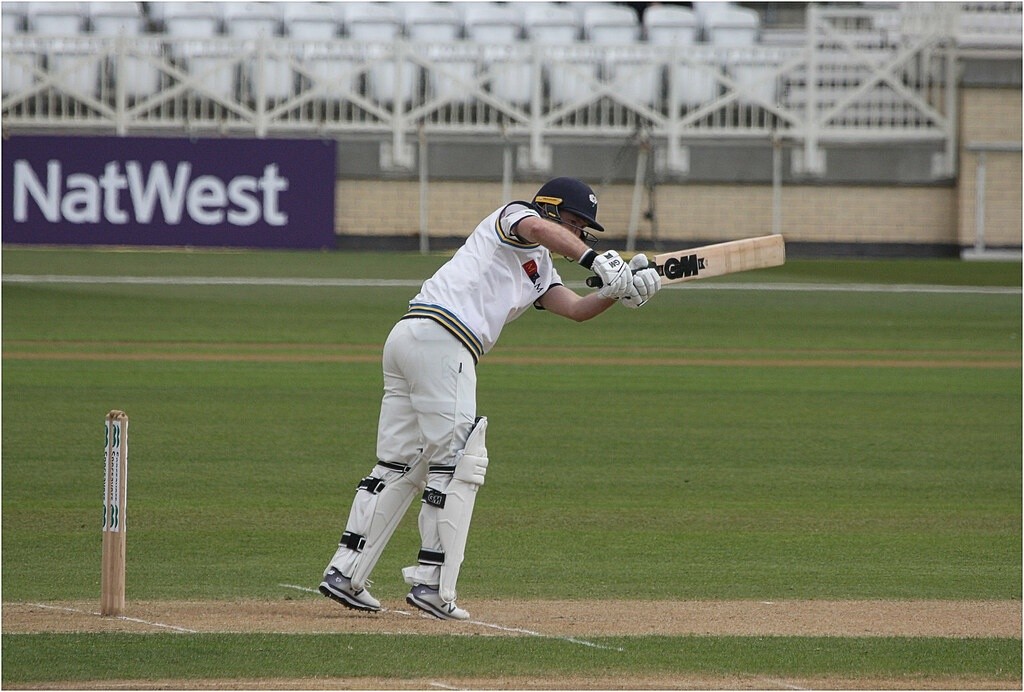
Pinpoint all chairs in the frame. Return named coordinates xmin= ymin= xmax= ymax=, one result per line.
xmin=1 ymin=1 xmax=790 ymax=130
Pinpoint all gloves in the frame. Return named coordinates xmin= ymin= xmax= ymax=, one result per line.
xmin=621 ymin=255 xmax=660 ymax=310
xmin=592 ymin=249 xmax=634 ymax=302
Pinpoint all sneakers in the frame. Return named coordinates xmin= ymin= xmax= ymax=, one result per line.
xmin=405 ymin=581 xmax=470 ymax=621
xmin=318 ymin=566 xmax=380 ymax=613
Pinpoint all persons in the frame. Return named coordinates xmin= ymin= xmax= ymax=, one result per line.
xmin=316 ymin=177 xmax=661 ymax=623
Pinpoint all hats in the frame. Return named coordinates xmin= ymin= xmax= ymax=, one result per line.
xmin=530 ymin=177 xmax=605 ymax=232
